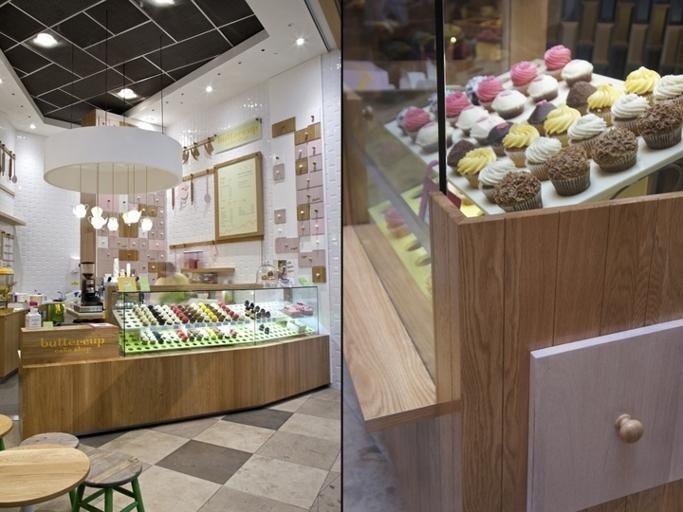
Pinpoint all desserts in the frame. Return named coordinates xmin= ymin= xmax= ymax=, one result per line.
xmin=396 ymin=44 xmax=683 ymax=212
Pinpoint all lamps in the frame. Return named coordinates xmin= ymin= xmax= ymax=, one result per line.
xmin=43 ymin=0 xmax=183 ymax=234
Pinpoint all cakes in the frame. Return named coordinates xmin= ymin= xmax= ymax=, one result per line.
xmin=281 ymin=301 xmax=313 ymax=317
xmin=385 ymin=208 xmax=411 ymax=237
xmin=133 ymin=300 xmax=271 ymax=346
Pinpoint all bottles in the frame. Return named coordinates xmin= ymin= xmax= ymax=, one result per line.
xmin=182 ymin=272 xmax=218 ymax=284
xmin=182 ymin=250 xmax=206 ymax=270
xmin=24 ymin=307 xmax=42 ymax=329
xmin=223 ymin=279 xmax=234 ymax=305
xmin=104 ymin=257 xmax=134 ymax=285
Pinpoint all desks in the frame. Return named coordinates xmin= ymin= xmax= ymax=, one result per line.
xmin=0 ymin=412 xmax=13 ymax=450
xmin=0 ymin=441 xmax=91 ymax=511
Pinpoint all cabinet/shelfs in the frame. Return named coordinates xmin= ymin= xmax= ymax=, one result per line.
xmin=0 ymin=211 xmax=49 ymax=384
xmin=343 ymin=0 xmax=683 ymax=512
xmin=112 ymin=283 xmax=320 ymax=358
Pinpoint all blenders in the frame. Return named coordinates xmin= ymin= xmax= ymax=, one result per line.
xmin=79 ymin=261 xmax=96 ymax=302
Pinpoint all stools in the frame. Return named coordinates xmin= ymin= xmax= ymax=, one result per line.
xmin=70 ymin=452 xmax=146 ymax=511
xmin=17 ymin=431 xmax=79 ymax=448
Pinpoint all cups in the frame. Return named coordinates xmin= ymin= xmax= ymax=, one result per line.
xmin=14 ymin=292 xmax=48 ymax=304
